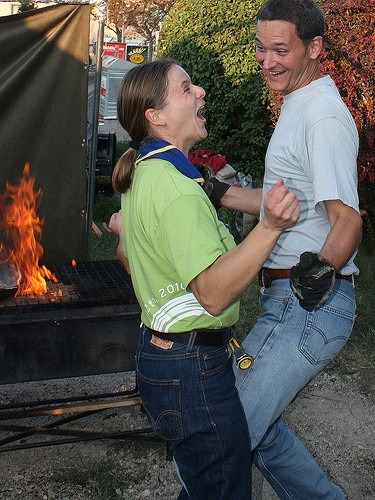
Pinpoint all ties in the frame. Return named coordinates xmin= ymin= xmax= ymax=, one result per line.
xmin=134 ymin=137 xmax=231 ymax=233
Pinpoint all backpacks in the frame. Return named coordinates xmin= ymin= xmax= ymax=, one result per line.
xmin=188 ymin=150 xmax=238 ymax=186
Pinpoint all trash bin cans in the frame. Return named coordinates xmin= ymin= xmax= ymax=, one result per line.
xmin=95 ymin=131 xmax=116 ymax=175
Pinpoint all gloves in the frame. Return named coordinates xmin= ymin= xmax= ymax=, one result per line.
xmin=289 ymin=252 xmax=335 ymax=311
xmin=198 ymin=177 xmax=231 ymax=212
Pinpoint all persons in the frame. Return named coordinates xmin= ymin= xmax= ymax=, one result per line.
xmin=110 ymin=60 xmax=301 ymax=500
xmin=172 ymin=0 xmax=363 ymax=500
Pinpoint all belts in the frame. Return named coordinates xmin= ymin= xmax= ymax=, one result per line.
xmin=257 ymin=267 xmax=353 ymax=288
xmin=143 ymin=323 xmax=234 ymax=347
